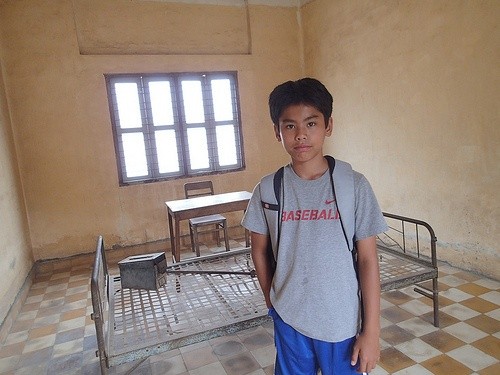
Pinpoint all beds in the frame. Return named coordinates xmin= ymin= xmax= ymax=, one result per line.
xmin=91 ymin=211 xmax=439 ymax=375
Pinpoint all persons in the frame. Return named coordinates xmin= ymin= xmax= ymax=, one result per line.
xmin=241 ymin=78 xmax=389 ymax=375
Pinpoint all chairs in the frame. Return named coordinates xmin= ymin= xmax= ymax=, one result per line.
xmin=184 ymin=180 xmax=230 ymax=257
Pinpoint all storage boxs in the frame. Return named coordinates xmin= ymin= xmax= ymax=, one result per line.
xmin=116 ymin=251 xmax=168 ymax=289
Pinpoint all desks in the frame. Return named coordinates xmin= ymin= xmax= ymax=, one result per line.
xmin=164 ymin=190 xmax=252 ymax=292
